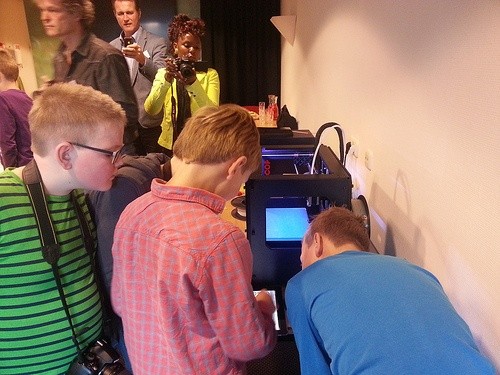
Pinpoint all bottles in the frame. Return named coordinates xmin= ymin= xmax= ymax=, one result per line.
xmin=268 ymin=95 xmax=279 ymax=120
xmin=259 ymin=102 xmax=265 ymax=123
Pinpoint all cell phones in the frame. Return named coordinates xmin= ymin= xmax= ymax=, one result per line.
xmin=124 ymin=36 xmax=134 ymax=47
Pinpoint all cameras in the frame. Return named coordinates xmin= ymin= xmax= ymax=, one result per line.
xmin=174 ymin=57 xmax=207 ymax=78
xmin=66 ymin=338 xmax=133 ymax=375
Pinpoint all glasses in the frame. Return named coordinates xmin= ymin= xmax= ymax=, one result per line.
xmin=65 ymin=141 xmax=125 ymax=164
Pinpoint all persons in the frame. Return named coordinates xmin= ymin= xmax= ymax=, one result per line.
xmin=0 ymin=47 xmax=33 ymax=170
xmin=284 ymin=200 xmax=496 ymax=375
xmin=0 ymin=82 xmax=128 ymax=375
xmin=110 ymin=103 xmax=276 ymax=375
xmin=106 ymin=0 xmax=172 ymax=156
xmin=142 ymin=13 xmax=220 ymax=159
xmin=32 ymin=0 xmax=140 ymax=144
